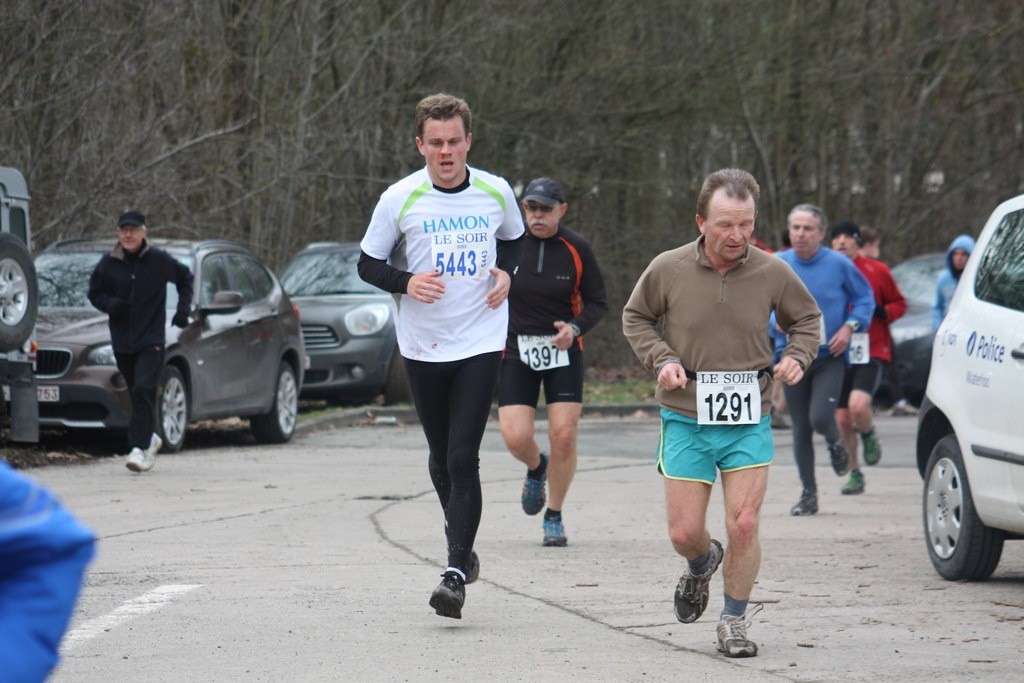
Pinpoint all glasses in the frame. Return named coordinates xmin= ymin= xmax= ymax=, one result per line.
xmin=525 ymin=202 xmax=552 ymax=212
xmin=119 ymin=226 xmax=137 ymax=233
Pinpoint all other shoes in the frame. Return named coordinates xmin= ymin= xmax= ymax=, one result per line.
xmin=892 ymin=401 xmax=919 ymax=416
xmin=771 ymin=403 xmax=791 ymax=429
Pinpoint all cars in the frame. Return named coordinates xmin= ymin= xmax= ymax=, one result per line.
xmin=279 ymin=242 xmax=413 ymax=408
xmin=870 ymin=252 xmax=951 ymax=408
xmin=916 ymin=194 xmax=1024 ymax=581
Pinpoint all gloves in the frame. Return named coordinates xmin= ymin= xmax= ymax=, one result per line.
xmin=172 ymin=312 xmax=188 ymax=328
xmin=873 ymin=304 xmax=886 ymax=320
xmin=107 ymin=297 xmax=131 ymax=316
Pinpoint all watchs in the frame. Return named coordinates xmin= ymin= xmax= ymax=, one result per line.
xmin=847 ymin=320 xmax=859 ymax=331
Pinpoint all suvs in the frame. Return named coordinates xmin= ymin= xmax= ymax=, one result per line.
xmin=3 ymin=236 xmax=307 ymax=456
xmin=0 ymin=166 xmax=38 ymax=383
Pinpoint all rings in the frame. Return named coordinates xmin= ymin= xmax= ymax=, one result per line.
xmin=791 ymin=373 xmax=795 ymax=378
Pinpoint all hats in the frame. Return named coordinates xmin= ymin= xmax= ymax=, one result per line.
xmin=526 ymin=177 xmax=564 ymax=207
xmin=118 ymin=211 xmax=145 ymax=227
xmin=830 ymin=221 xmax=863 ymax=247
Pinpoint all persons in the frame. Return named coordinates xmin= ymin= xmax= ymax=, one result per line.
xmin=832 ymin=221 xmax=918 ymax=493
xmin=622 ymin=170 xmax=820 ymax=657
xmin=770 ymin=205 xmax=875 ymax=516
xmin=497 ymin=178 xmax=610 ymax=546
xmin=88 ymin=211 xmax=194 ymax=472
xmin=751 ymin=230 xmax=793 ymax=428
xmin=0 ymin=462 xmax=95 ymax=683
xmin=932 ymin=235 xmax=975 ymax=330
xmin=357 ymin=94 xmax=526 ymax=619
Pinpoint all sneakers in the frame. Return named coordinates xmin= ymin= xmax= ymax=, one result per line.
xmin=673 ymin=540 xmax=723 ymax=623
xmin=842 ymin=471 xmax=863 ymax=494
xmin=445 ymin=522 xmax=479 ymax=583
xmin=862 ymin=425 xmax=881 ymax=465
xmin=716 ymin=602 xmax=765 ymax=658
xmin=125 ymin=447 xmax=144 ymax=473
xmin=540 ymin=515 xmax=567 ymax=547
xmin=827 ymin=438 xmax=850 ymax=475
xmin=522 ymin=451 xmax=548 ymax=515
xmin=790 ymin=488 xmax=818 ymax=516
xmin=142 ymin=433 xmax=163 ymax=471
xmin=430 ymin=571 xmax=465 ymax=619
